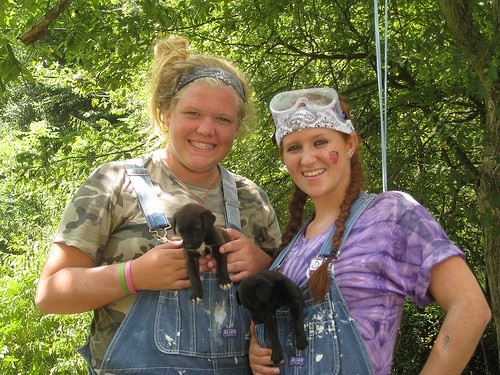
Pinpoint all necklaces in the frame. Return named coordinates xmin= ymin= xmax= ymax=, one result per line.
xmin=165 ymin=162 xmax=221 ymax=210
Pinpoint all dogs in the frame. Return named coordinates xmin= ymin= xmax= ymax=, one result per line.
xmin=236 ymin=270 xmax=309 ymax=364
xmin=172 ymin=204 xmax=234 ymax=304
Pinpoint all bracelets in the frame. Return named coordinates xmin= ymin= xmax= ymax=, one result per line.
xmin=125 ymin=260 xmax=140 ymax=295
xmin=117 ymin=261 xmax=132 ymax=296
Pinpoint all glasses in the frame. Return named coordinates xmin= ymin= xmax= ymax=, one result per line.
xmin=270 ymin=87 xmax=345 ymax=123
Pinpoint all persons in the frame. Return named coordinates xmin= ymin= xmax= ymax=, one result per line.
xmin=237 ymin=85 xmax=495 ymax=375
xmin=25 ymin=31 xmax=292 ymax=374
